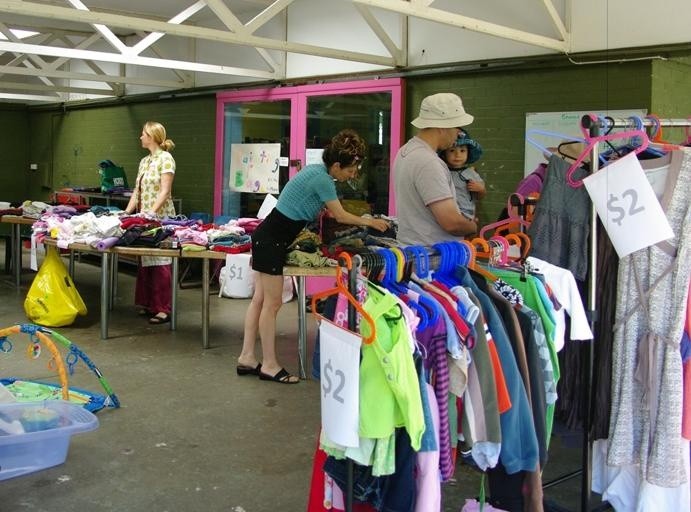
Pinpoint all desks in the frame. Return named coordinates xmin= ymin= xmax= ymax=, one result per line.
xmin=1 ymin=213 xmax=181 ymax=341
xmin=181 ymin=249 xmax=351 ymax=381
xmin=53 ymin=189 xmax=183 ymax=212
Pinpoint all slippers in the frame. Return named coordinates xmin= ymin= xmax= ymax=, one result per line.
xmin=259 ymin=361 xmax=301 ymax=386
xmin=237 ymin=361 xmax=262 ymax=377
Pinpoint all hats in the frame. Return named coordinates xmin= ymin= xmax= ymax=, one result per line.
xmin=437 ymin=126 xmax=482 ymax=165
xmin=410 ymin=92 xmax=475 ymax=131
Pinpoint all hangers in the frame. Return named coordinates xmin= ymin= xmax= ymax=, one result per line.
xmin=309 ymin=190 xmax=532 ymax=344
xmin=524 ymin=114 xmax=691 ymax=188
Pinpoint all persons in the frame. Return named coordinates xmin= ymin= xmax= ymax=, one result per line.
xmin=234 ymin=127 xmax=390 ymax=384
xmin=437 ymin=126 xmax=487 ymax=220
xmin=124 ymin=121 xmax=177 ymax=324
xmin=393 ymin=92 xmax=478 ymax=250
xmin=335 ymin=144 xmax=388 ymax=215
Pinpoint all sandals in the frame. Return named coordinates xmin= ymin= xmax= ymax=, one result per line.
xmin=139 ymin=307 xmax=148 ymax=316
xmin=150 ymin=313 xmax=170 ymax=325
